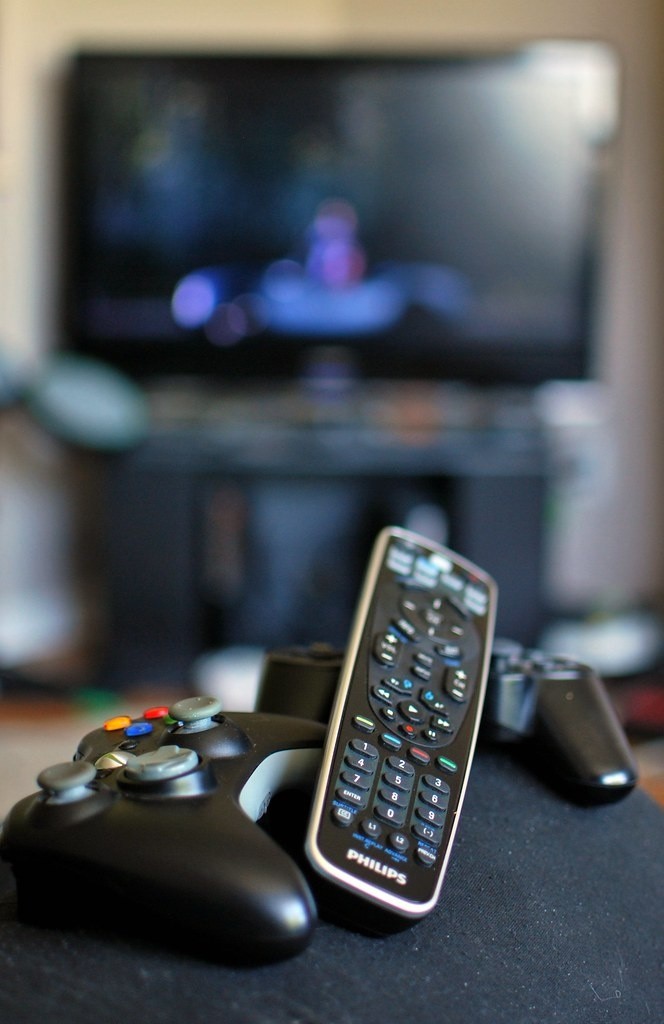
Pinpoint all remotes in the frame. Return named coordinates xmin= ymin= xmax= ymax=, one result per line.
xmin=305 ymin=526 xmax=500 ymax=919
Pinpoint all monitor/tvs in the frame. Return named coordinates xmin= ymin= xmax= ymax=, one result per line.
xmin=60 ymin=35 xmax=616 ymax=389
